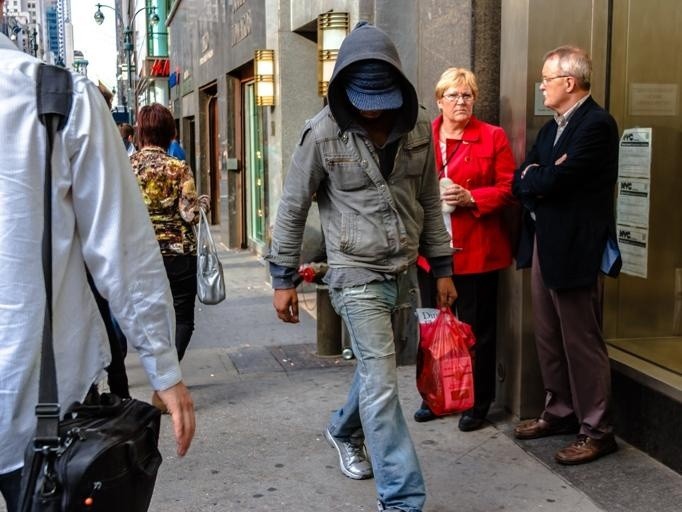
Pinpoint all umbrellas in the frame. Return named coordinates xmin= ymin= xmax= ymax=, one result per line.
xmin=554 ymin=436 xmax=618 ymax=465
xmin=513 ymin=417 xmax=580 ymax=440
xmin=458 ymin=415 xmax=484 ymax=431
xmin=414 ymin=408 xmax=435 ymax=422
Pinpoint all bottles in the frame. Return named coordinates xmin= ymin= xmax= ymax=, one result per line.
xmin=344 ymin=61 xmax=403 ymax=111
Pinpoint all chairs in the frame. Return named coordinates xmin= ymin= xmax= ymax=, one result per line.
xmin=322 ymin=428 xmax=373 ymax=479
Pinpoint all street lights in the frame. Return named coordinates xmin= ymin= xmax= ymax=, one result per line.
xmin=16 ymin=62 xmax=163 ymax=512
xmin=197 ymin=207 xmax=226 ymax=305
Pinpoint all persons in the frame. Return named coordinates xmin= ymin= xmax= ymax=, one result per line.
xmin=110 ymin=101 xmax=212 ymax=414
xmin=0 ymin=0 xmax=196 ymax=512
xmin=267 ymin=18 xmax=458 ymax=512
xmin=412 ymin=67 xmax=519 ymax=432
xmin=117 ymin=123 xmax=136 ymax=155
xmin=512 ymin=44 xmax=623 ymax=464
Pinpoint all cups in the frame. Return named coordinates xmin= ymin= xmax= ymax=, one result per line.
xmin=540 ymin=75 xmax=571 ymax=87
xmin=442 ymin=90 xmax=473 ymax=101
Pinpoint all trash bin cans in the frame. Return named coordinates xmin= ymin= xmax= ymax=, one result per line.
xmin=469 ymin=197 xmax=476 ymax=204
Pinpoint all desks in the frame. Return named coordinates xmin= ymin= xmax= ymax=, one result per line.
xmin=94 ymin=1 xmax=158 ymax=134
xmin=11 ymin=27 xmax=30 ymax=56
